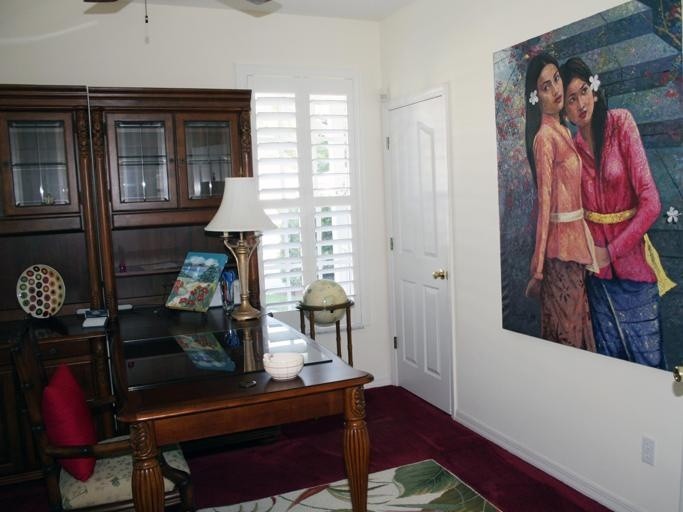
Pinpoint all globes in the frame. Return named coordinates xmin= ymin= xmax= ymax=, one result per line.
xmin=295 ymin=279 xmax=354 ymax=368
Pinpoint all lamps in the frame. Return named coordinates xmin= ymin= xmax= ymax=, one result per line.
xmin=237 ymin=324 xmax=257 ymax=374
xmin=205 ymin=177 xmax=278 ymax=321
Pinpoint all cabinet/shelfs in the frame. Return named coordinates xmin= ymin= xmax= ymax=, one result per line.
xmin=0 ymin=85 xmax=260 ymax=485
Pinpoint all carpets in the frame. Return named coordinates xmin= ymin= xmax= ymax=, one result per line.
xmin=196 ymin=458 xmax=503 ymax=512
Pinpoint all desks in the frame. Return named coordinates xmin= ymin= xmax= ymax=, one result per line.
xmin=112 ymin=301 xmax=373 ymax=512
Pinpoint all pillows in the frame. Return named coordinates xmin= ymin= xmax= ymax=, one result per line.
xmin=43 ymin=364 xmax=99 ymax=482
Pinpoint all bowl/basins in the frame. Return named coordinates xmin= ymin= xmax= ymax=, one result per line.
xmin=262 ymin=352 xmax=303 ymax=380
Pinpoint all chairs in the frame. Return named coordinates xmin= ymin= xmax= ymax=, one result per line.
xmin=10 ymin=331 xmax=193 ymax=511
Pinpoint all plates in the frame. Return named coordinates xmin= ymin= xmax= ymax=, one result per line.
xmin=16 ymin=263 xmax=66 ymax=319
